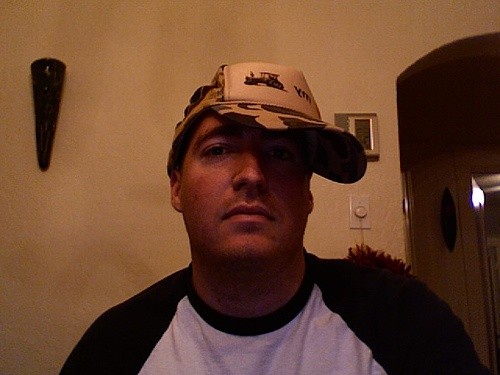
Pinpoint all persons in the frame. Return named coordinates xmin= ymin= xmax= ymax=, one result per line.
xmin=56 ymin=61 xmax=492 ymax=375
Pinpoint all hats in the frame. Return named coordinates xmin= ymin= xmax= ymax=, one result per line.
xmin=167 ymin=61 xmax=367 ymax=186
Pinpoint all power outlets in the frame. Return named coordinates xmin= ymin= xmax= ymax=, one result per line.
xmin=350 ymin=197 xmax=371 ymax=229
